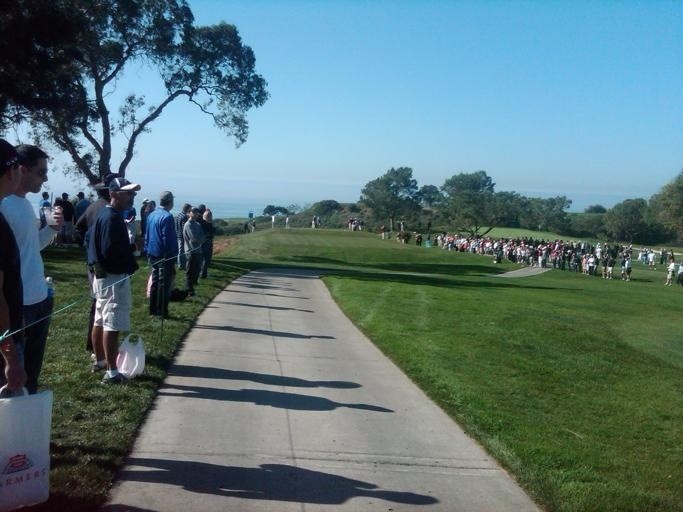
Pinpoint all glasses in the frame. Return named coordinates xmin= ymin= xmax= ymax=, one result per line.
xmin=27 ymin=167 xmax=48 ymax=177
xmin=117 ymin=190 xmax=137 ymax=195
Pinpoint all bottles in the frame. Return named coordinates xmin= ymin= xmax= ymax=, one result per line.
xmin=45 ymin=276 xmax=55 ymax=298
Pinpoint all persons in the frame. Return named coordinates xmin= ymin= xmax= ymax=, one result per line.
xmin=0 ymin=144 xmax=64 ymax=393
xmin=637 ymin=246 xmax=673 ymax=271
xmin=38 ymin=189 xmax=213 ymax=318
xmin=284 ymin=215 xmax=289 ymax=229
xmin=432 ymin=232 xmax=632 ymax=282
xmin=312 ymin=215 xmax=321 ymax=229
xmin=72 ymin=173 xmax=124 ymax=355
xmin=663 ymin=257 xmax=683 ymax=287
xmin=381 ymin=225 xmax=386 ymax=239
xmin=416 ymin=233 xmax=422 ymax=247
xmin=82 ymin=177 xmax=140 ymax=385
xmin=0 ymin=137 xmax=27 ymax=396
xmin=347 ymin=217 xmax=364 ymax=231
xmin=251 ymin=219 xmax=256 ymax=233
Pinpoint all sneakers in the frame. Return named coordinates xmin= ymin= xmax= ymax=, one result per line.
xmin=100 ymin=372 xmax=129 ymax=385
xmin=90 ymin=360 xmax=106 ymax=373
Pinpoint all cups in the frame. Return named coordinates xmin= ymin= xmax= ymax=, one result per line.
xmin=42 ymin=207 xmax=61 ymax=225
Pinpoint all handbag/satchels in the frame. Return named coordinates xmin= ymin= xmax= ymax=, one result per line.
xmin=0 ymin=383 xmax=54 ymax=511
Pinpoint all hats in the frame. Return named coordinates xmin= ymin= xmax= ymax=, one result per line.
xmin=188 ymin=206 xmax=199 ymax=213
xmin=160 ymin=191 xmax=174 ymax=202
xmin=0 ymin=138 xmax=19 ymax=169
xmin=108 ymin=177 xmax=141 ymax=192
